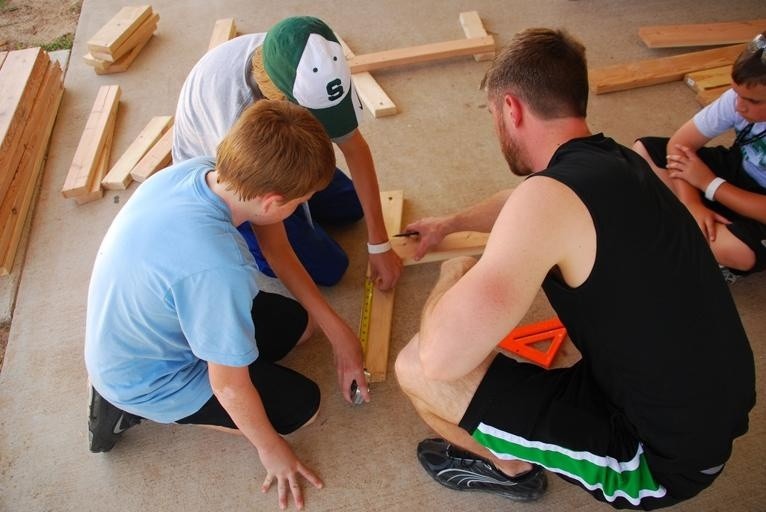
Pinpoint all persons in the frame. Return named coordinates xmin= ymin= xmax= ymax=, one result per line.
xmin=393 ymin=28 xmax=757 ymax=512
xmin=80 ymin=98 xmax=338 ymax=510
xmin=631 ymin=30 xmax=766 ymax=286
xmin=170 ymin=16 xmax=403 ymax=406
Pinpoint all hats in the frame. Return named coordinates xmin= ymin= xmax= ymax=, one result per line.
xmin=261 ymin=15 xmax=357 ymax=140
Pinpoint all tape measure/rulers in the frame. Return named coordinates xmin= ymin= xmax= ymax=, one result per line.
xmin=350 ymin=279 xmax=374 ymax=404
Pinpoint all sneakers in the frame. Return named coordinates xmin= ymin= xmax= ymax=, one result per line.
xmin=88 ymin=380 xmax=141 ymax=453
xmin=417 ymin=438 xmax=547 ymax=502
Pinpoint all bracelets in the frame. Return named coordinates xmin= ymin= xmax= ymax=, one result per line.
xmin=367 ymin=240 xmax=392 ymax=255
xmin=705 ymin=176 xmax=727 ymax=202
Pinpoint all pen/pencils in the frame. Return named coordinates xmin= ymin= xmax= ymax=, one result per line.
xmin=392 ymin=231 xmax=419 ymax=237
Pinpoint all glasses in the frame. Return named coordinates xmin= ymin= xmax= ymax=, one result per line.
xmin=748 ymin=32 xmax=766 ymax=68
